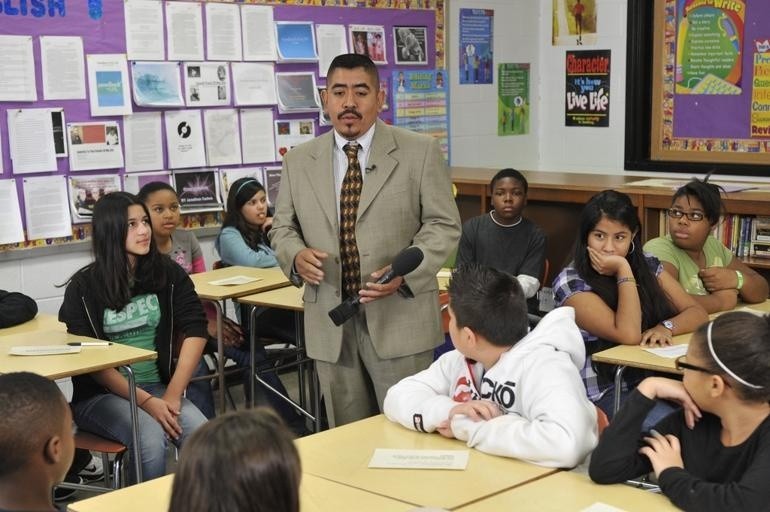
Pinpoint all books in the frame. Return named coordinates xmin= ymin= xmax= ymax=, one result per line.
xmin=659 ymin=211 xmax=770 ymax=256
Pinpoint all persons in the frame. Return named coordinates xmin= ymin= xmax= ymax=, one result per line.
xmin=352 ymin=32 xmax=383 ymax=61
xmin=219 ymin=177 xmax=297 ymax=343
xmin=1 ymin=371 xmax=75 ymax=512
xmin=58 ymin=192 xmax=210 ymax=484
xmin=573 ymin=0 xmax=585 ymax=45
xmin=643 ymin=181 xmax=768 ymax=312
xmin=383 ymin=261 xmax=600 ymax=478
xmin=105 ymin=128 xmax=118 ymax=145
xmin=267 ymin=52 xmax=464 ymax=429
xmin=463 ymin=53 xmax=490 ymax=83
xmin=71 ymin=126 xmax=81 ymax=144
xmin=435 ymin=71 xmax=445 ymax=89
xmin=459 ymin=169 xmax=546 ymax=315
xmin=589 ymin=311 xmax=769 ymax=511
xmin=502 ymin=107 xmax=526 ymax=132
xmin=553 ymin=190 xmax=708 ymax=433
xmin=395 ymin=71 xmax=406 ymax=92
xmin=170 ymin=409 xmax=300 ymax=512
xmin=140 ymin=181 xmax=300 ymax=436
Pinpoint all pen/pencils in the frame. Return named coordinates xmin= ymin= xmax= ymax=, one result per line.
xmin=66 ymin=341 xmax=113 ymax=346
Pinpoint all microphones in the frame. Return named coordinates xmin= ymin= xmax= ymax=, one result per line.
xmin=328 ymin=247 xmax=425 ymax=327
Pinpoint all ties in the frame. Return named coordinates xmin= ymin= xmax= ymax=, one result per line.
xmin=339 ymin=144 xmax=363 ymax=301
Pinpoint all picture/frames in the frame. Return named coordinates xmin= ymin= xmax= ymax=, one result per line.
xmin=623 ymin=0 xmax=769 ymax=178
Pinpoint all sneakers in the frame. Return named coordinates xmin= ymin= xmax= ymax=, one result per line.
xmin=53 ymin=476 xmax=83 ymax=502
xmin=78 ymin=456 xmax=124 ymax=485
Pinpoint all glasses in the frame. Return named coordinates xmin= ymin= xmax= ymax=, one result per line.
xmin=667 ymin=208 xmax=706 ymax=222
xmin=675 ymin=354 xmax=733 ymax=389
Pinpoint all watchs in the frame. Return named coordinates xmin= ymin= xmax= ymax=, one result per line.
xmin=660 ymin=320 xmax=675 ymax=335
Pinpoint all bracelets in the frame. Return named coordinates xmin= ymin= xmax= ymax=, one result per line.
xmin=618 ymin=278 xmax=637 ymax=284
xmin=736 ymin=271 xmax=743 ymax=289
